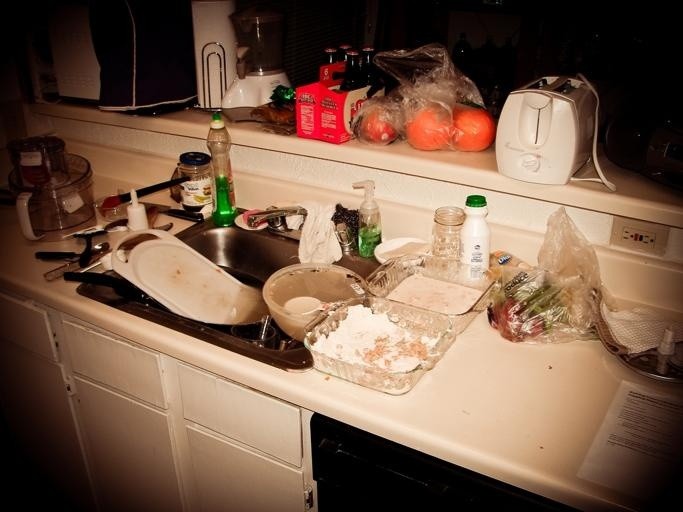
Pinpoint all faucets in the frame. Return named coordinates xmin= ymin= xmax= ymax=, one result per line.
xmin=246 ymin=206 xmax=307 ymax=241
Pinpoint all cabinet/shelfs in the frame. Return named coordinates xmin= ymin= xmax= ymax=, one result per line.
xmin=0 ymin=285 xmax=179 ymax=510
xmin=176 ymin=361 xmax=309 ymax=512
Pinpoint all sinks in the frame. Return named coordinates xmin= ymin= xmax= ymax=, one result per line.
xmin=114 ymin=225 xmax=307 ymax=351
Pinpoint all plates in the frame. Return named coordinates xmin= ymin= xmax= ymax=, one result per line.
xmin=374 ymin=237 xmax=428 ymax=267
xmin=304 ymin=294 xmax=455 ymax=397
xmin=365 ymin=252 xmax=499 ymax=337
xmin=235 ymin=214 xmax=268 ymax=231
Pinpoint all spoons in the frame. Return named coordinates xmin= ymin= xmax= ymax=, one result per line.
xmin=35 ymin=242 xmax=110 ymax=261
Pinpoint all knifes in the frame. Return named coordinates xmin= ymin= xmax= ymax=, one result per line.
xmin=146 ymin=203 xmax=203 ymax=222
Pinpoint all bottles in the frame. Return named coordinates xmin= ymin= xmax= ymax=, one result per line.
xmin=326 ymin=48 xmax=337 ymax=63
xmin=461 ymin=195 xmax=490 ymax=282
xmin=178 ymin=152 xmax=214 ymax=212
xmin=341 ymin=50 xmax=363 ymax=91
xmin=207 ymin=114 xmax=240 ymax=227
xmin=341 ymin=42 xmax=352 ymax=59
xmin=361 ymin=47 xmax=374 ymax=85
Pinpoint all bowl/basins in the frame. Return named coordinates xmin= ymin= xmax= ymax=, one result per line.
xmin=7 ymin=137 xmax=66 ymax=188
xmin=8 ymin=154 xmax=96 ymax=240
xmin=263 ymin=262 xmax=368 ymax=341
xmin=96 ymin=195 xmax=130 ymax=221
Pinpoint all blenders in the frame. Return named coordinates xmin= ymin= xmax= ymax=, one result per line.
xmin=221 ymin=0 xmax=292 ymax=122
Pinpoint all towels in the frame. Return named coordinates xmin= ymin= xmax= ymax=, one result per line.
xmin=593 ymin=290 xmax=683 ymax=355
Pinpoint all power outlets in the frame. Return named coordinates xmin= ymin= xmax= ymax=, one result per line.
xmin=610 ymin=216 xmax=671 ymax=257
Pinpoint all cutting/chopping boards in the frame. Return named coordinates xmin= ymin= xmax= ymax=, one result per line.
xmin=112 ymin=229 xmax=261 ymax=324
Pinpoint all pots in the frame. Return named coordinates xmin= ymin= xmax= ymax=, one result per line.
xmin=63 ymin=264 xmax=265 ymax=339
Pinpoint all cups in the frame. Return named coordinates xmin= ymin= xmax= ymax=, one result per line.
xmin=285 ymin=297 xmax=325 ymax=321
xmin=231 ymin=324 xmax=276 ymax=350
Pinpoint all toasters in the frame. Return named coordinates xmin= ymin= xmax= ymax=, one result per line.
xmin=494 ymin=76 xmax=600 ymax=186
